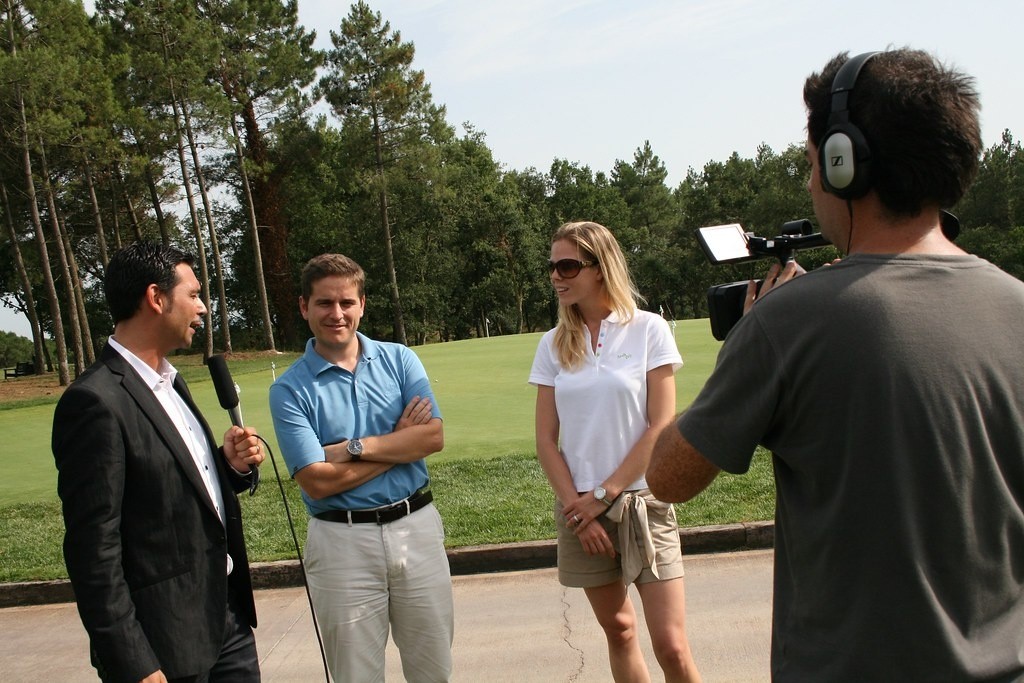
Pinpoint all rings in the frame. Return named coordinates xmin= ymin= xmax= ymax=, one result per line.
xmin=255 ymin=444 xmax=261 ymax=453
xmin=574 ymin=515 xmax=580 ymax=524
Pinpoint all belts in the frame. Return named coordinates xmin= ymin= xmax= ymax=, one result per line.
xmin=312 ymin=489 xmax=433 ymax=525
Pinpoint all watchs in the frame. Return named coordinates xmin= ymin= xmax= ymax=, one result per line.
xmin=346 ymin=439 xmax=363 ymax=462
xmin=593 ymin=485 xmax=612 ymax=507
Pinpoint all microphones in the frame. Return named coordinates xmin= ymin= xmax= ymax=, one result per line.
xmin=206 ymin=353 xmax=259 ymax=481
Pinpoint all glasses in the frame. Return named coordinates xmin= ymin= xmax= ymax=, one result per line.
xmin=546 ymin=258 xmax=599 ymax=280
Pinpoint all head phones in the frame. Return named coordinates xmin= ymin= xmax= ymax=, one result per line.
xmin=816 ymin=50 xmax=889 ymax=201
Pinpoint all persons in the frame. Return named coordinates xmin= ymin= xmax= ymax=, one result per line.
xmin=528 ymin=221 xmax=702 ymax=683
xmin=268 ymin=252 xmax=453 ymax=683
xmin=51 ymin=243 xmax=268 ymax=683
xmin=646 ymin=48 xmax=1023 ymax=683
